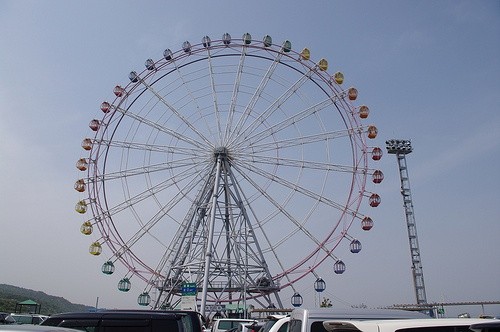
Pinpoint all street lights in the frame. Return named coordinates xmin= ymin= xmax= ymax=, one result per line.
xmin=385 ymin=139 xmax=428 ymax=306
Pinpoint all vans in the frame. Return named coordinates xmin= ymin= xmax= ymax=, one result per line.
xmin=0 ymin=311 xmax=500 ymax=332
